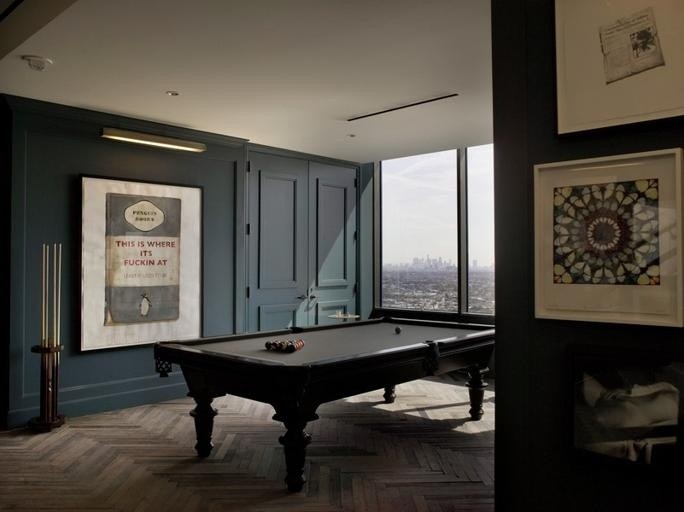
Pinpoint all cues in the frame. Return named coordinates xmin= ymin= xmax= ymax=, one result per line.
xmin=41 ymin=243 xmax=62 ymax=425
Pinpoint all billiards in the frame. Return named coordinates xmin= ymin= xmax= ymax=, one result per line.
xmin=395 ymin=327 xmax=402 ymax=334
xmin=265 ymin=338 xmax=304 ymax=353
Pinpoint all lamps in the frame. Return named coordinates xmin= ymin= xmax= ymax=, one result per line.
xmin=100 ymin=127 xmax=207 ymax=154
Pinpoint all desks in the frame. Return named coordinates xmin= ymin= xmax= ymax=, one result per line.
xmin=154 ymin=317 xmax=497 ymax=492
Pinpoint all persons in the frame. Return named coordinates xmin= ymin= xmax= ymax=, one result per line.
xmin=576 ymin=364 xmax=679 ymax=430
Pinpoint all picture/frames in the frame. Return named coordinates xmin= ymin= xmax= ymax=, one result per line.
xmin=76 ymin=172 xmax=204 ymax=354
xmin=550 ymin=0 xmax=683 ymax=138
xmin=532 ymin=145 xmax=684 ymax=329
